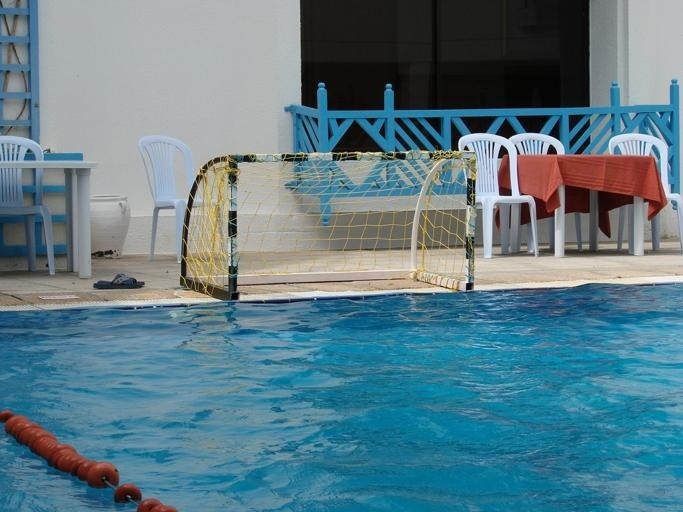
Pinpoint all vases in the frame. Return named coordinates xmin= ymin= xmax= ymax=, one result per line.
xmin=89 ymin=195 xmax=131 ymax=260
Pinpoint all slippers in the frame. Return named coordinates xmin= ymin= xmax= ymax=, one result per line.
xmin=94 ymin=273 xmax=144 ymax=288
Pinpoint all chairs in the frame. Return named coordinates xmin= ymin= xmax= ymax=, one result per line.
xmin=457 ymin=133 xmax=683 ymax=259
xmin=137 ymin=135 xmax=226 ymax=265
xmin=0 ymin=135 xmax=56 ymax=276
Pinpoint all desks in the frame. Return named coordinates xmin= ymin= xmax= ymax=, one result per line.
xmin=0 ymin=161 xmax=101 ymax=278
